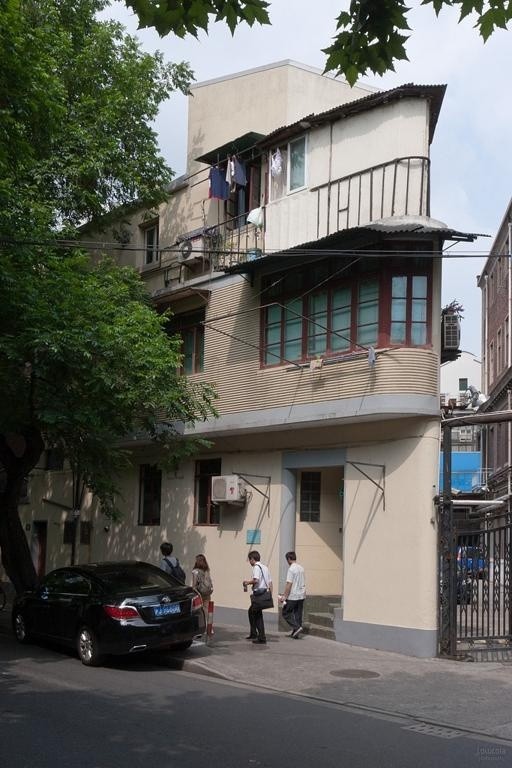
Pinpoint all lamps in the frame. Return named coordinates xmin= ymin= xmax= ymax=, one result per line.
xmin=446 ymin=385 xmax=494 ymax=412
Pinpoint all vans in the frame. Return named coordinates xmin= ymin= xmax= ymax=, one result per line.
xmin=456 ymin=545 xmax=487 ymax=575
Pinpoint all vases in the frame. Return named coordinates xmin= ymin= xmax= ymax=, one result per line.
xmin=246 ymin=247 xmax=261 ymax=262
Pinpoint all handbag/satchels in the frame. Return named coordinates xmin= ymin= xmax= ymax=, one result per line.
xmin=250 ymin=590 xmax=273 ymax=609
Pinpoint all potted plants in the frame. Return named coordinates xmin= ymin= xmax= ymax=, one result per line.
xmin=442 ymin=298 xmax=465 ymax=319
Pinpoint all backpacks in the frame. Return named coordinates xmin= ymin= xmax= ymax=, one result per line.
xmin=164 ymin=557 xmax=186 ymax=584
xmin=195 ymin=568 xmax=214 ymax=596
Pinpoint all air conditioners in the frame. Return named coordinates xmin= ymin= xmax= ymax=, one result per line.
xmin=178 ymin=225 xmax=213 ymax=270
xmin=442 ymin=314 xmax=460 ymax=350
xmin=212 ymin=475 xmax=244 ymax=506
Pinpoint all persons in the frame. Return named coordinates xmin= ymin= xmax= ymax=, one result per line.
xmin=242 ymin=551 xmax=274 ymax=645
xmin=192 ymin=554 xmax=214 ymax=641
xmin=279 ymin=552 xmax=306 ymax=640
xmin=157 ymin=542 xmax=182 ymax=584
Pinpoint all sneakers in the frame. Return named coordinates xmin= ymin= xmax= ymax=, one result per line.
xmin=286 ymin=625 xmax=303 ymax=640
xmin=246 ymin=634 xmax=257 ymax=640
xmin=253 ymin=637 xmax=267 ymax=644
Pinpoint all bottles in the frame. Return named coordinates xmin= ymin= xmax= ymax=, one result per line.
xmin=277 ymin=595 xmax=287 ymax=606
xmin=243 ymin=581 xmax=248 ymax=592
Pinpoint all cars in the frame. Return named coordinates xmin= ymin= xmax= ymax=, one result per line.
xmin=7 ymin=557 xmax=209 ymax=669
xmin=440 ymin=553 xmax=475 ymax=606
